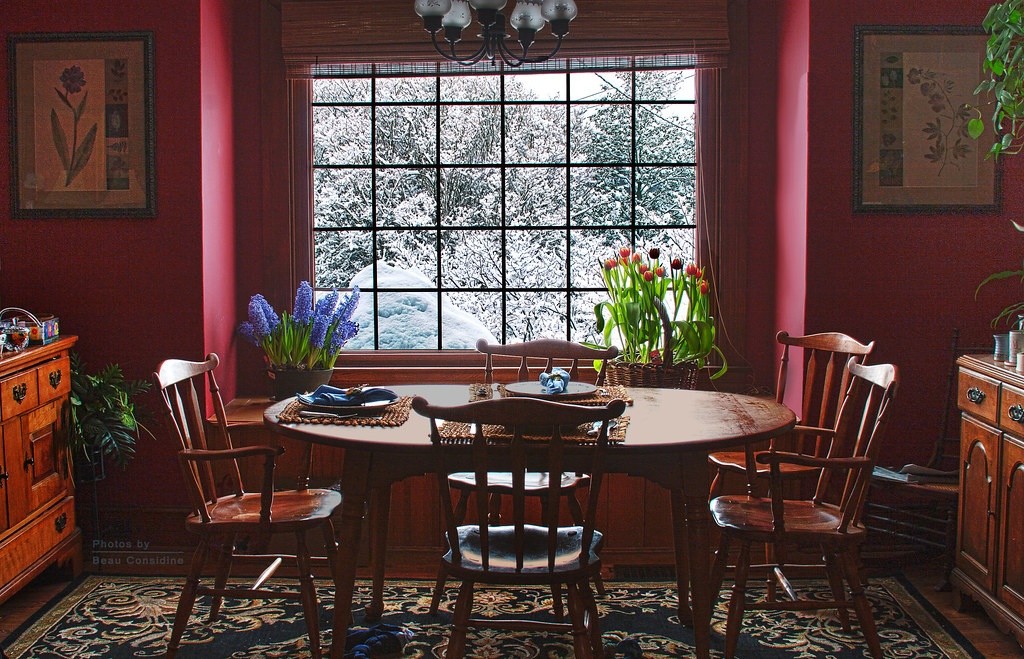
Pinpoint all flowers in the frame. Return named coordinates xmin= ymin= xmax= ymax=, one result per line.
xmin=237 ymin=280 xmax=362 ymax=369
xmin=577 ymin=247 xmax=727 ymax=380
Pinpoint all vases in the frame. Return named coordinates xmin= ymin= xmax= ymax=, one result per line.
xmin=266 ymin=365 xmax=334 ymax=402
xmin=604 ymin=353 xmax=702 ymax=390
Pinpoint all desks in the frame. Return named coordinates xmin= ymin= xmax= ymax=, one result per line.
xmin=263 ymin=385 xmax=796 ymax=659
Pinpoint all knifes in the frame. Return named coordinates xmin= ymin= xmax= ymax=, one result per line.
xmin=592 ymin=421 xmax=602 ymax=435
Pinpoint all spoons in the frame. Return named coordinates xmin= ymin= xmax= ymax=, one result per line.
xmin=607 ymin=422 xmax=616 ymax=437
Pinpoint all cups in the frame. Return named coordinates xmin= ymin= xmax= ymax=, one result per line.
xmin=1009 ymin=330 xmax=1024 ymax=363
xmin=993 ymin=334 xmax=1009 ymax=361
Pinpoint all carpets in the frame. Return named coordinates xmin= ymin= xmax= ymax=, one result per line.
xmin=0 ymin=572 xmax=987 ymax=659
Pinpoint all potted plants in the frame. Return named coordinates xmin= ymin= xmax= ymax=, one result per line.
xmin=61 ymin=347 xmax=156 ymax=489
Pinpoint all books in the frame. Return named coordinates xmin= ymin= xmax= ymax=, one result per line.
xmin=873 ymin=464 xmax=958 ymax=484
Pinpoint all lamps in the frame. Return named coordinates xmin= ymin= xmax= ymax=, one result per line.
xmin=414 ymin=0 xmax=578 ymax=67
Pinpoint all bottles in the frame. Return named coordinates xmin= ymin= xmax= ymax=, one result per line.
xmin=2 ymin=317 xmax=29 ymax=351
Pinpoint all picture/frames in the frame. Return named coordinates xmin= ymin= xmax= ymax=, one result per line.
xmin=851 ymin=23 xmax=1004 ymax=215
xmin=6 ymin=31 xmax=159 ymax=218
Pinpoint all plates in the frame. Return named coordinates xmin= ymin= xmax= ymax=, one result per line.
xmin=298 ymin=394 xmax=400 ymax=413
xmin=505 ymin=382 xmax=597 ymax=397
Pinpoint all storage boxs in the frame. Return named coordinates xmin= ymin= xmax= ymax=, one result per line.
xmin=1 ymin=315 xmax=60 ymax=347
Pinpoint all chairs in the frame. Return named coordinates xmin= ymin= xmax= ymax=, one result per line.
xmin=409 ymin=391 xmax=625 ymax=658
xmin=152 ymin=351 xmax=354 ymax=659
xmin=705 ymin=330 xmax=877 ymax=632
xmin=863 ymin=328 xmax=995 ymax=591
xmin=427 ymin=339 xmax=620 ymax=622
xmin=711 ymin=357 xmax=901 ymax=659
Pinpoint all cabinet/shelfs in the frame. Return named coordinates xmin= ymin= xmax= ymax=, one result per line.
xmin=0 ymin=335 xmax=83 ymax=605
xmin=949 ymin=351 xmax=1024 ymax=651
